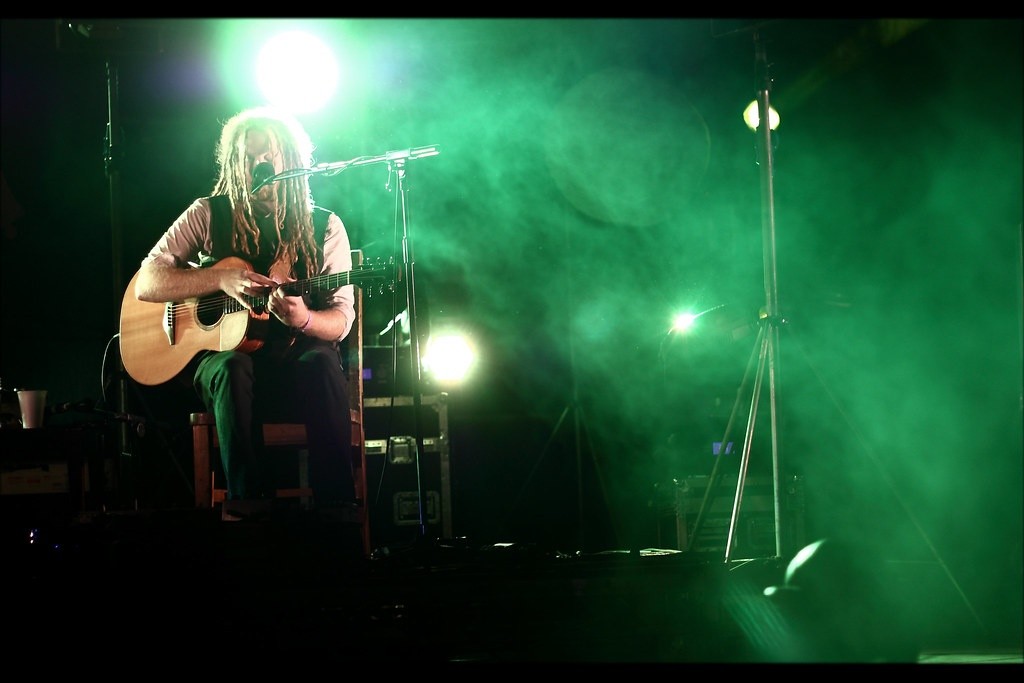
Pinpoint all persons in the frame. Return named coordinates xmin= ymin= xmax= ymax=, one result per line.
xmin=134 ymin=107 xmax=356 ymax=517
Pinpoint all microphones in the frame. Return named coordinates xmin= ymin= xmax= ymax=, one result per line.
xmin=251 ymin=162 xmax=274 ymax=193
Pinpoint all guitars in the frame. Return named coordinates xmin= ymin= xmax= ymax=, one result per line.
xmin=119 ymin=255 xmax=402 ymax=387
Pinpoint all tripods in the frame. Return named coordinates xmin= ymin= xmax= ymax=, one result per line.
xmin=509 ymin=215 xmax=627 ymax=554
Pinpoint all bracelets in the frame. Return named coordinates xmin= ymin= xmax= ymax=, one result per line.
xmin=300 ymin=312 xmax=313 ymax=331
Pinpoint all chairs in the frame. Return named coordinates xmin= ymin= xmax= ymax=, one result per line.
xmin=190 ymin=250 xmax=369 ymax=554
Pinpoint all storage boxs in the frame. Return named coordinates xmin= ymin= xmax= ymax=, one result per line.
xmin=653 ymin=474 xmax=805 ymax=558
xmin=363 ymin=393 xmax=452 ymax=541
